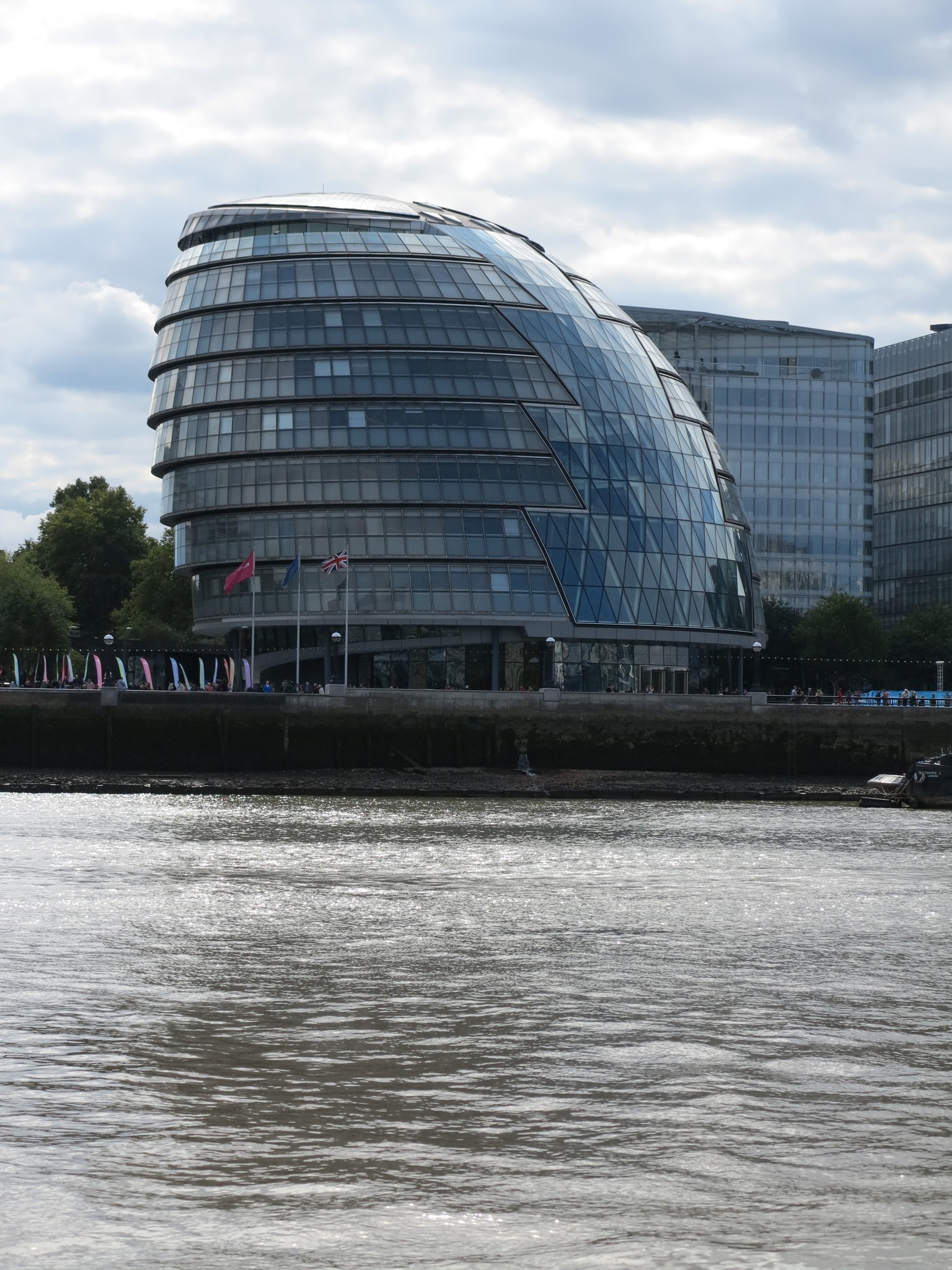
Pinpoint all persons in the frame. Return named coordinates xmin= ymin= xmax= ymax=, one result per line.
xmin=262 ymin=678 xmax=952 ymax=707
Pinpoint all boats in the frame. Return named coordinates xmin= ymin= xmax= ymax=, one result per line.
xmin=905 ymin=743 xmax=952 ymax=809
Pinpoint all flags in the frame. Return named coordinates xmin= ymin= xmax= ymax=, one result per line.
xmin=13 ymin=653 xmax=252 ymax=691
xmin=282 ymin=553 xmax=300 ymax=587
xmin=321 ymin=549 xmax=347 ymax=575
xmin=224 ymin=550 xmax=255 ymax=595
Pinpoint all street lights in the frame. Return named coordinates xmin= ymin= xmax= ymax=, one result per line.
xmin=544 ymin=637 xmax=557 ymax=688
xmin=751 ymin=642 xmax=762 ymax=692
xmin=102 ymin=634 xmax=115 ymax=687
xmin=329 ymin=632 xmax=341 ymax=685
